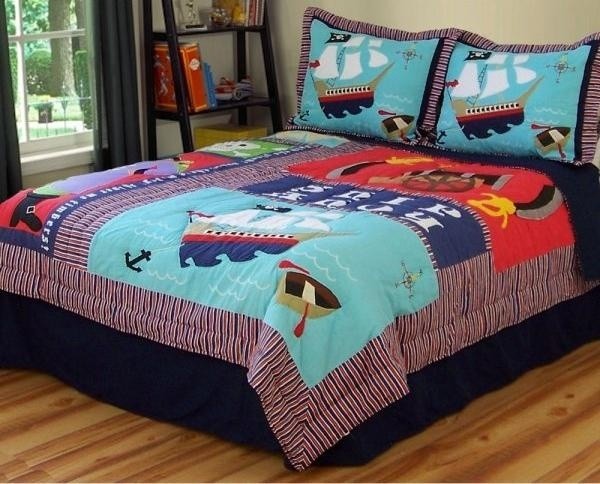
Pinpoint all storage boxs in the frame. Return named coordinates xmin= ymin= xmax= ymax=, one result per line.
xmin=194 ymin=124 xmax=267 ymax=149
xmin=155 ymin=40 xmax=209 ymax=113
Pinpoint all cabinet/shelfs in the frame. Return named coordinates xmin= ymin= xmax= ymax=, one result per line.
xmin=145 ymin=0 xmax=281 ymax=157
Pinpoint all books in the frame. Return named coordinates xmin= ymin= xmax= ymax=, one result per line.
xmin=211 ymin=0 xmax=264 ymax=28
xmin=203 ymin=60 xmax=216 ymax=108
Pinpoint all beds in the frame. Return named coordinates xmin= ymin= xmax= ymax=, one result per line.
xmin=0 ymin=130 xmax=600 ymax=470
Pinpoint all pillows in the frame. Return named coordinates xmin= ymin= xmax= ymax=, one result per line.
xmin=286 ymin=8 xmax=454 ymax=144
xmin=429 ymin=27 xmax=600 ymax=166
